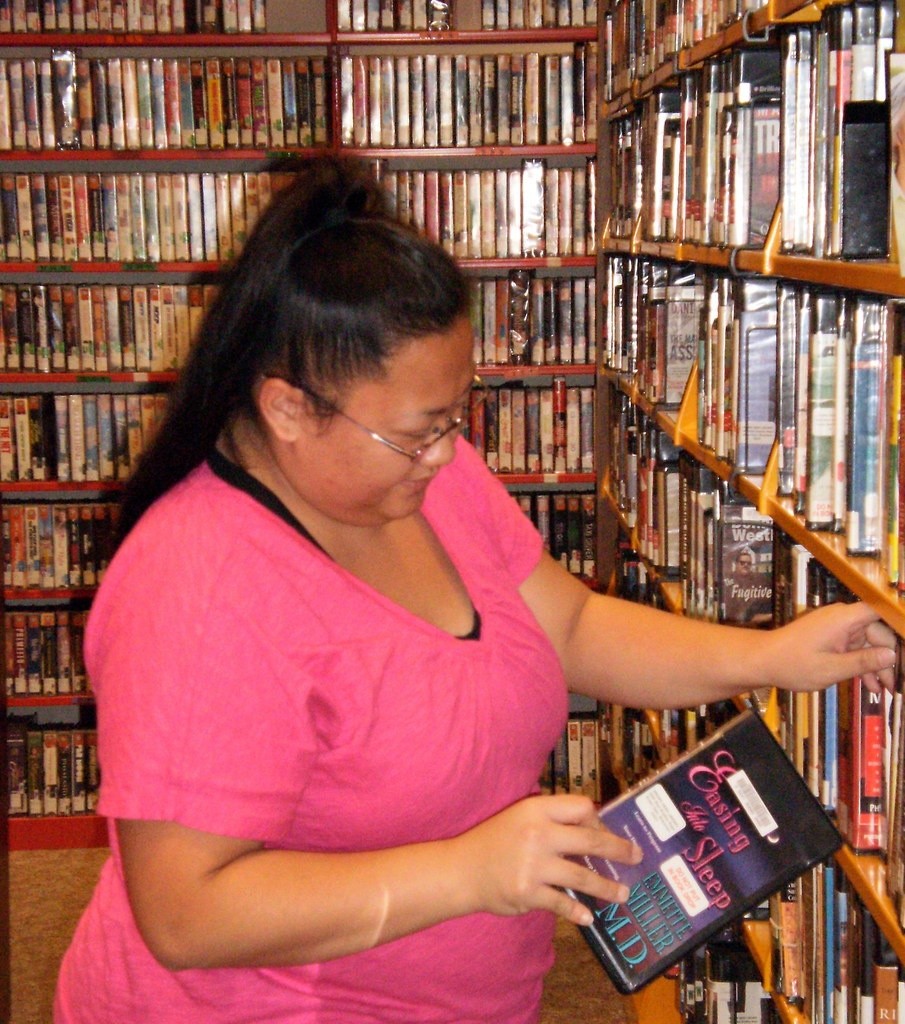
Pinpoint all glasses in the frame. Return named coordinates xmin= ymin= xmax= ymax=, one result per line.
xmin=265 ymin=365 xmax=488 ymax=460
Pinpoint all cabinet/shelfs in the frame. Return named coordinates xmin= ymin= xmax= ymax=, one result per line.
xmin=0 ymin=0 xmax=905 ymax=1024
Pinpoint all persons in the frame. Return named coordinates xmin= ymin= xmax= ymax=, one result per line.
xmin=52 ymin=157 xmax=905 ymax=1024
xmin=727 ymin=553 xmax=774 ymax=624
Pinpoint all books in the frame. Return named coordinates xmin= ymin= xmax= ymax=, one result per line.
xmin=0 ymin=0 xmax=905 ymax=1024
